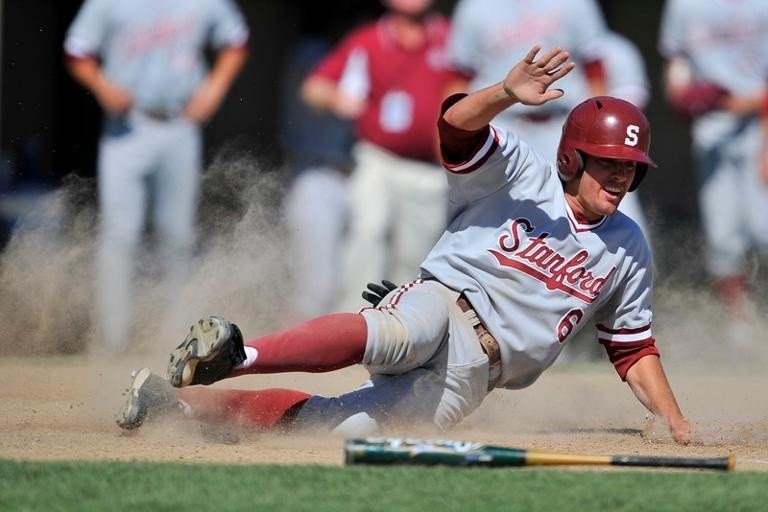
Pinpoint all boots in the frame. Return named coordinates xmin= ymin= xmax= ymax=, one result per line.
xmin=165 ymin=314 xmax=249 ymax=388
xmin=114 ymin=367 xmax=177 ymax=429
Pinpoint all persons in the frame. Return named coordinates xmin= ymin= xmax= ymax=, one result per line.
xmin=60 ymin=1 xmax=254 ymax=367
xmin=113 ymin=41 xmax=703 ymax=449
xmin=279 ymin=1 xmax=767 ymax=322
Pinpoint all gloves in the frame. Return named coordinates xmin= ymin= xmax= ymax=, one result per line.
xmin=359 ymin=279 xmax=397 ymax=307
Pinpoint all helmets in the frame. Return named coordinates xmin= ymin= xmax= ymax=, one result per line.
xmin=554 ymin=94 xmax=657 ymax=193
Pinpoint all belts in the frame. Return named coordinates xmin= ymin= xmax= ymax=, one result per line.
xmin=454 ymin=295 xmax=512 ymax=394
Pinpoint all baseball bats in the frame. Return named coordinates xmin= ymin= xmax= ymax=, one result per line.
xmin=343 ymin=436 xmax=737 ymax=472
